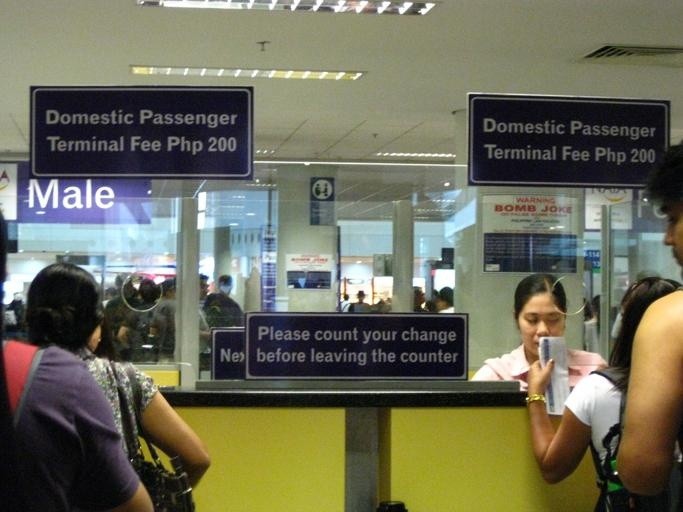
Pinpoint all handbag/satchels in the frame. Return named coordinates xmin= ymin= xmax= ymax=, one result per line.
xmin=130 ymin=456 xmax=194 ymax=512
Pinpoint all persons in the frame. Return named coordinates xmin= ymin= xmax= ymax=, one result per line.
xmin=466 ymin=271 xmax=611 ymax=389
xmin=0 ymin=207 xmax=154 ymax=511
xmin=614 ymin=139 xmax=683 ymax=497
xmin=583 ymin=295 xmax=600 ymax=353
xmin=339 ymin=286 xmax=455 ymax=313
xmin=0 ymin=272 xmax=244 ymax=368
xmin=523 ymin=276 xmax=682 ymax=512
xmin=26 ymin=262 xmax=212 ymax=510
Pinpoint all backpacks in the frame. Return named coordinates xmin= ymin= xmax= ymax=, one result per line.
xmin=588 ymin=370 xmax=682 ymax=512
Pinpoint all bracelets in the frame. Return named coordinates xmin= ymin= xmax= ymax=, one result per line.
xmin=524 ymin=394 xmax=546 ymax=408
